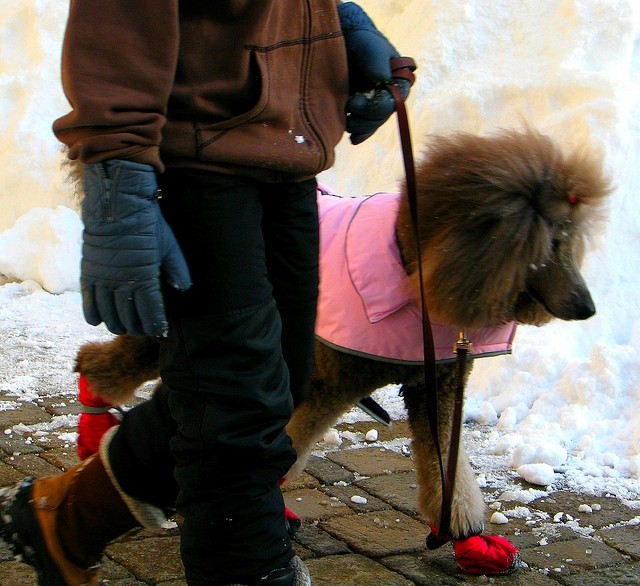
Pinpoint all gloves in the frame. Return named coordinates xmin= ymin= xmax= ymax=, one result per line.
xmin=79 ymin=161 xmax=192 ymax=340
xmin=336 ymin=1 xmax=411 ymax=146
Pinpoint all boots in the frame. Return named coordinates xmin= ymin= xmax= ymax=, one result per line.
xmin=0 ymin=423 xmax=179 ymax=586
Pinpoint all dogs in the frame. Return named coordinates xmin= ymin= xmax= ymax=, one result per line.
xmin=60 ymin=111 xmax=620 ymax=576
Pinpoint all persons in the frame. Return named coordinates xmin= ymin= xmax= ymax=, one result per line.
xmin=0 ymin=0 xmax=416 ymax=586
